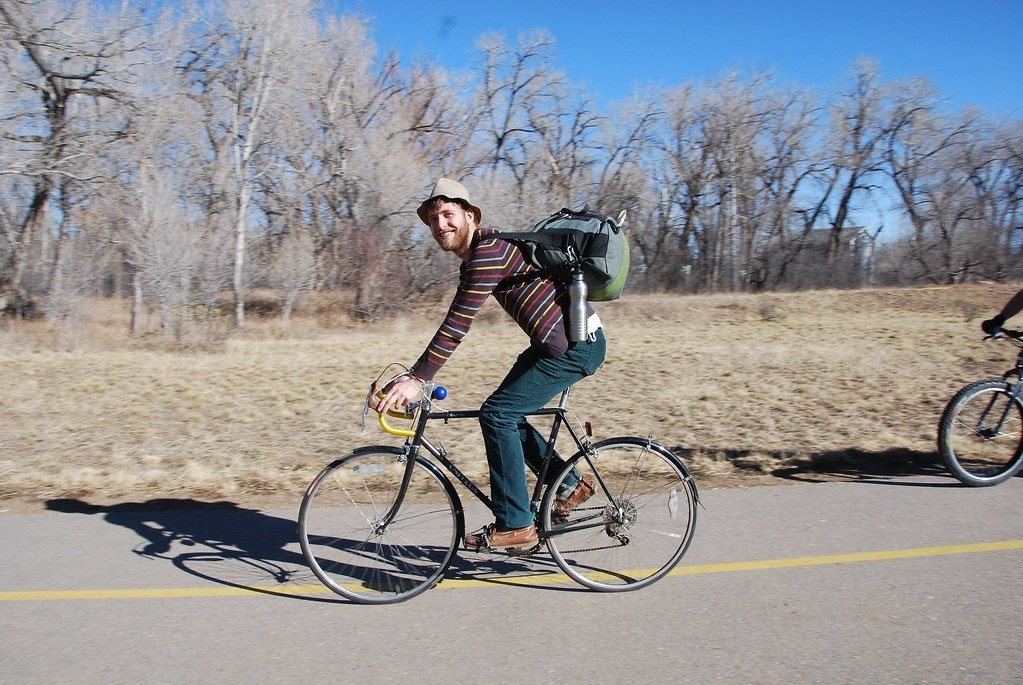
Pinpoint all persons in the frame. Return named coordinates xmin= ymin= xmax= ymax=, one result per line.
xmin=378 ymin=178 xmax=606 ymax=549
xmin=982 ymin=289 xmax=1023 ymax=335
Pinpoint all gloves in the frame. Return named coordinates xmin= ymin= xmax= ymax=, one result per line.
xmin=982 ymin=315 xmax=1005 ymax=334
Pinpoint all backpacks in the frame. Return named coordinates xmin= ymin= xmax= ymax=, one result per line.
xmin=473 ymin=203 xmax=630 ymax=302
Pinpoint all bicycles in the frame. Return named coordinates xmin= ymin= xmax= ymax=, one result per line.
xmin=297 ymin=381 xmax=700 ymax=605
xmin=937 ymin=329 xmax=1023 ymax=488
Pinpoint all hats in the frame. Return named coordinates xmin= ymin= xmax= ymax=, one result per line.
xmin=417 ymin=178 xmax=481 ymax=227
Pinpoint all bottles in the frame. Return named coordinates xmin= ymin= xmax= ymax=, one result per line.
xmin=567 ymin=263 xmax=588 ymax=341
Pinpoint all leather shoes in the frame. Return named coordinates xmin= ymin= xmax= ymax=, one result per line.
xmin=464 ymin=524 xmax=538 ymax=549
xmin=553 ymin=476 xmax=598 ymax=522
